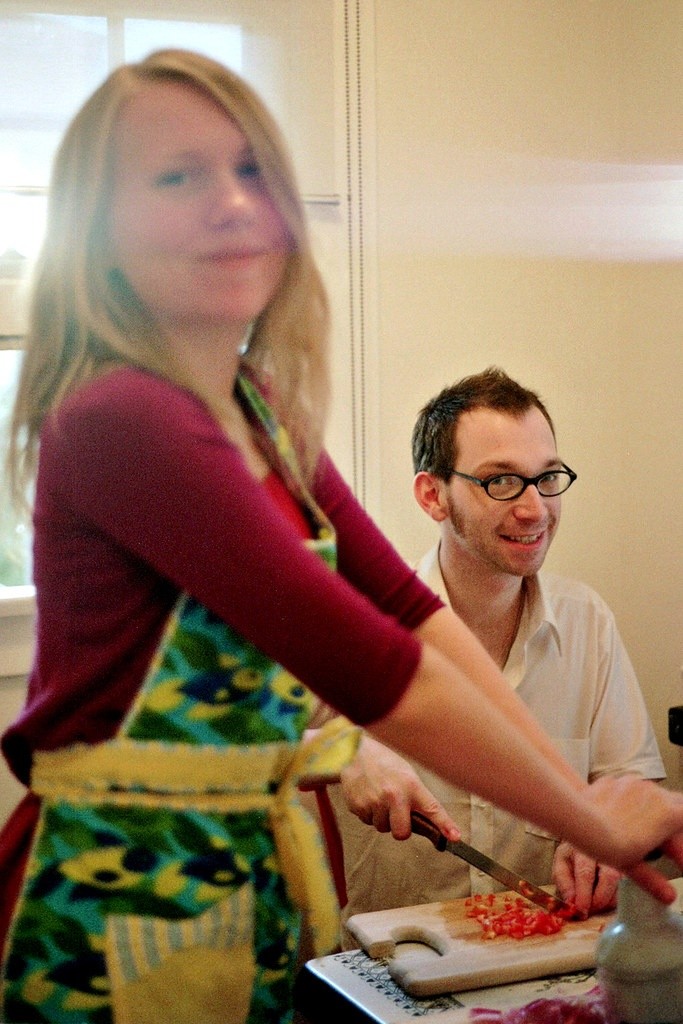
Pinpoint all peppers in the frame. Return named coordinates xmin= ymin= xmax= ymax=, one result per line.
xmin=466 ymin=880 xmax=568 ymax=939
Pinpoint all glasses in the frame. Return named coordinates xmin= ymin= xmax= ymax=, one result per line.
xmin=449 ymin=462 xmax=577 ymax=502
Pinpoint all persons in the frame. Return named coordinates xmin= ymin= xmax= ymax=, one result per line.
xmin=282 ymin=365 xmax=669 ymax=952
xmin=0 ymin=50 xmax=683 ymax=1024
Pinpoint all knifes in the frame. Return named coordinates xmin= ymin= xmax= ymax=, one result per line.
xmin=406 ymin=813 xmax=591 ymax=921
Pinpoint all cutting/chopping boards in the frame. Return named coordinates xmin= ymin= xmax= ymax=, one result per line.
xmin=346 ymin=881 xmax=615 ymax=996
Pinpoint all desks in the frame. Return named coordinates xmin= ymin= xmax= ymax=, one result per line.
xmin=296 ymin=877 xmax=683 ymax=1024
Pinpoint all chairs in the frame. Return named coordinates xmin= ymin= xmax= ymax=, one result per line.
xmin=297 ymin=775 xmax=350 ymax=909
